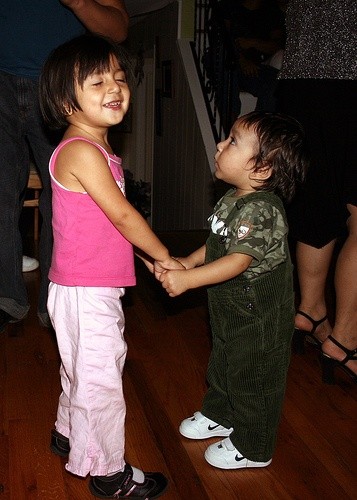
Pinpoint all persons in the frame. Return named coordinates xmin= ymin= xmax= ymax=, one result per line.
xmin=268 ymin=0 xmax=357 ymax=385
xmin=203 ymin=1 xmax=285 ymax=140
xmin=37 ymin=31 xmax=188 ymax=500
xmin=0 ymin=1 xmax=130 ymax=328
xmin=159 ymin=107 xmax=312 ymax=469
xmin=22 ymin=256 xmax=39 ymax=272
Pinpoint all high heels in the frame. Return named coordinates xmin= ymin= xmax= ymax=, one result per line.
xmin=320 ymin=335 xmax=357 ymax=384
xmin=291 ymin=309 xmax=328 ymax=355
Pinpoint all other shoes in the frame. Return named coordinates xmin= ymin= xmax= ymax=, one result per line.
xmin=0 ymin=309 xmax=20 ymax=333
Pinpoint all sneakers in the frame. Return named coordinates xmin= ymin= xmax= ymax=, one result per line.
xmin=22 ymin=256 xmax=39 ymax=272
xmin=179 ymin=411 xmax=233 ymax=439
xmin=204 ymin=436 xmax=272 ymax=469
xmin=89 ymin=461 xmax=168 ymax=500
xmin=51 ymin=429 xmax=70 ymax=456
